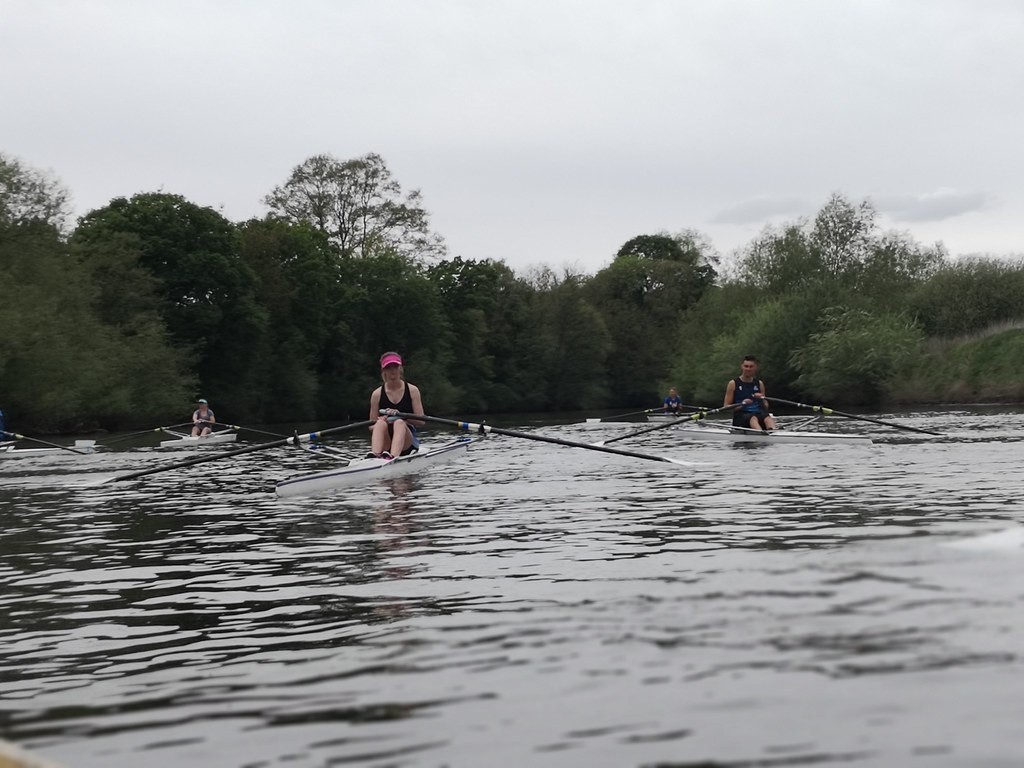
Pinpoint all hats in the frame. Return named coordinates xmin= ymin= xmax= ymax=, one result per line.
xmin=381 ymin=354 xmax=403 ymax=369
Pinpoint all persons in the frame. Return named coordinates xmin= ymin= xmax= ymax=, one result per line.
xmin=365 ymin=352 xmax=425 ymax=460
xmin=722 ymin=356 xmax=784 ymax=431
xmin=663 ymin=388 xmax=682 ymax=415
xmin=191 ymin=399 xmax=215 ymax=437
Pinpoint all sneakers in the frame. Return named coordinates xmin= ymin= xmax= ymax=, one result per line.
xmin=367 ymin=451 xmax=381 ymax=459
xmin=382 ymin=451 xmax=395 ymax=459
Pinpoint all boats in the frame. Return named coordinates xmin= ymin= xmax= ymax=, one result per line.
xmin=160 ymin=434 xmax=238 ymax=447
xmin=0 ymin=441 xmax=96 ymax=459
xmin=645 ymin=412 xmax=701 ymax=421
xmin=275 ymin=438 xmax=469 ymax=494
xmin=672 ymin=424 xmax=874 ymax=447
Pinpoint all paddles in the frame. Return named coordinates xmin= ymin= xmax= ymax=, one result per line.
xmin=1 ymin=428 xmax=84 ymax=457
xmin=74 ymin=421 xmax=196 ymax=446
xmin=588 ymin=399 xmax=750 ymax=449
xmin=585 ymin=406 xmax=669 ymax=424
xmin=379 ymin=407 xmax=720 ymax=470
xmin=752 ymin=392 xmax=946 ymax=438
xmin=204 ymin=421 xmax=291 ymax=440
xmin=102 ymin=418 xmax=376 ymax=484
xmin=681 ymin=406 xmax=713 ymax=412
